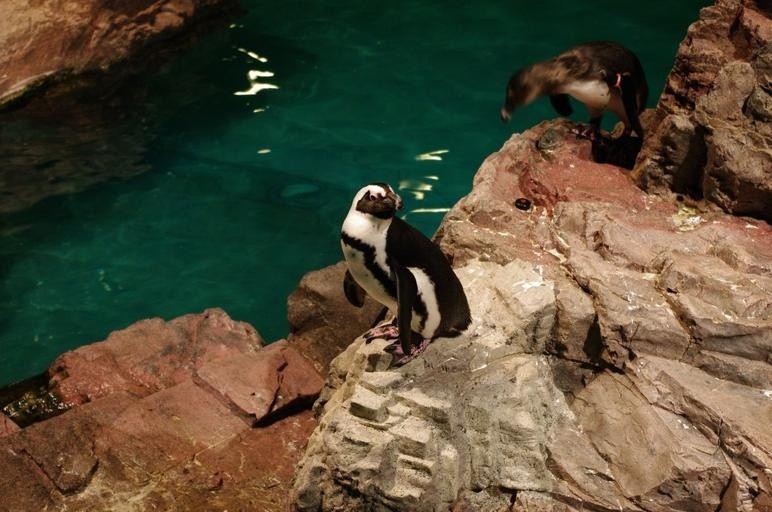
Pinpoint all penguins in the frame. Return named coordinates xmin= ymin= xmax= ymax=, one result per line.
xmin=340 ymin=182 xmax=471 ymax=357
xmin=501 ymin=44 xmax=648 ymax=142
